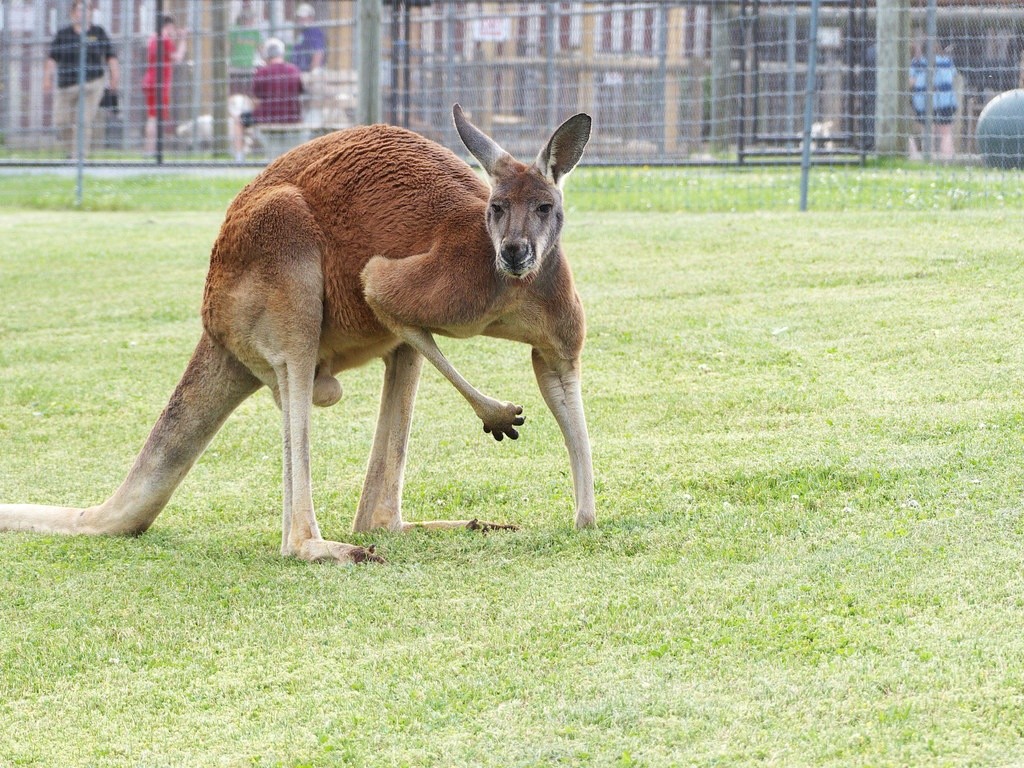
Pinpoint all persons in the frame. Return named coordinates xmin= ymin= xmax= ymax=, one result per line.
xmin=140 ymin=13 xmax=185 ymax=158
xmin=234 ymin=4 xmax=326 ymax=156
xmin=908 ymin=39 xmax=957 ymax=157
xmin=43 ymin=0 xmax=120 ymax=160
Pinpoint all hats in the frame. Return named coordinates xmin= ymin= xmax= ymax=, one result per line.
xmin=296 ymin=4 xmax=316 ymax=18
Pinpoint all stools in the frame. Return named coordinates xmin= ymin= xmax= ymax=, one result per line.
xmin=253 ymin=125 xmax=308 ymax=163
